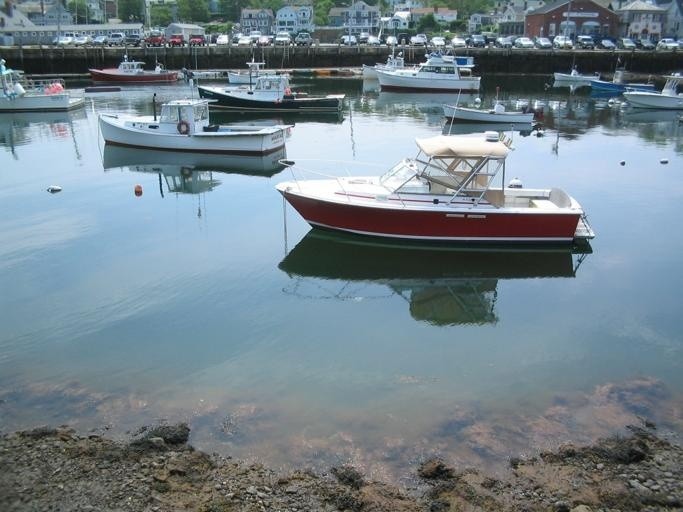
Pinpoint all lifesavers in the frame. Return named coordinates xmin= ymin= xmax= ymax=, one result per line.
xmin=180 ymin=167 xmax=193 ymax=178
xmin=177 ymin=120 xmax=190 ymax=135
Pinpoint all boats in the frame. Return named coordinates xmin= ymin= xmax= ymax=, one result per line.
xmin=275 ymin=131 xmax=597 ymax=240
